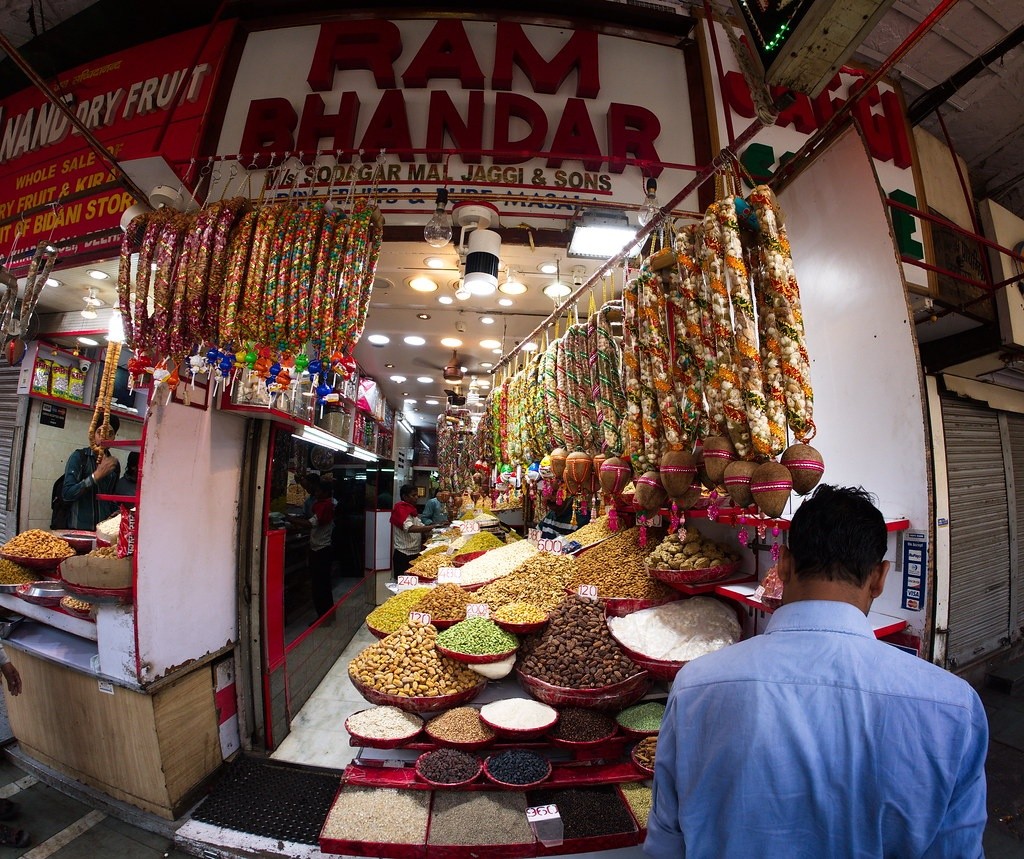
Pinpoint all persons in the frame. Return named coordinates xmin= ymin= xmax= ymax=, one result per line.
xmin=300 ymin=473 xmax=337 ymax=520
xmin=642 ymin=482 xmax=987 ymax=859
xmin=533 ymin=493 xmax=592 ymax=541
xmin=0 ymin=644 xmax=32 ymax=850
xmin=421 ymin=488 xmax=450 ymax=524
xmin=289 ymin=482 xmax=337 ymax=625
xmin=115 ymin=451 xmax=140 ymax=509
xmin=389 ymin=485 xmax=441 ymax=581
xmin=62 ymin=414 xmax=120 ymax=533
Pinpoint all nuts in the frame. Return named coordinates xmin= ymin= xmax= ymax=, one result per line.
xmin=349 ymin=506 xmax=737 ymax=784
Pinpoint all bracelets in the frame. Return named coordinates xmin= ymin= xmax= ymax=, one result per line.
xmin=91 ymin=473 xmax=98 ymax=485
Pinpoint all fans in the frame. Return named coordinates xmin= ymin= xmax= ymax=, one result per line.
xmin=0 ymin=299 xmax=39 ymax=346
xmin=424 ymin=385 xmax=486 ymax=407
xmin=411 ymin=350 xmax=492 ymax=381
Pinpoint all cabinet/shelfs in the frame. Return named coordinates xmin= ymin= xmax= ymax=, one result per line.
xmin=17 ymin=338 xmax=150 ymax=424
xmin=0 ymin=364 xmax=241 ymax=823
xmin=657 ymin=505 xmax=911 ymax=646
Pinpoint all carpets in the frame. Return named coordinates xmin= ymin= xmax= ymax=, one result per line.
xmin=191 ymin=754 xmax=345 ymax=847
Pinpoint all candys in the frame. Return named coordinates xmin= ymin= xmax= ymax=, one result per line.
xmin=121 ymin=202 xmax=383 ymax=419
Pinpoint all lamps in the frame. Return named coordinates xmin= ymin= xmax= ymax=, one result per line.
xmin=398 ymin=417 xmax=415 ymax=435
xmin=80 ymin=287 xmax=104 ymax=320
xmin=292 ymin=430 xmax=380 ymax=463
xmin=566 ymin=206 xmax=651 ymax=262
xmin=451 ymin=201 xmax=502 ymax=296
xmin=419 ymin=439 xmax=430 ymax=451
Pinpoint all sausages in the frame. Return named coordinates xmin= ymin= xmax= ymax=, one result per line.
xmin=20 ymin=240 xmax=59 ymax=338
xmin=88 ymin=341 xmax=122 ymax=464
xmin=0 ymin=266 xmax=18 ymax=356
